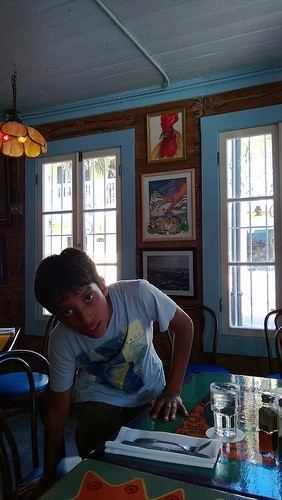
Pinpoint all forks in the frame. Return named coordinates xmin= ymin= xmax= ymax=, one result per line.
xmin=133 ymin=437 xmax=212 ymax=454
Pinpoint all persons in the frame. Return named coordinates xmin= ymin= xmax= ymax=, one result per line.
xmin=16 ymin=248 xmax=194 ymax=499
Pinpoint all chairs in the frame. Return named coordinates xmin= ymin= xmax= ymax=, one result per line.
xmin=0 ymin=314 xmax=85 ymax=500
xmin=164 ymin=305 xmax=230 ymax=378
xmin=265 ymin=308 xmax=282 ymax=379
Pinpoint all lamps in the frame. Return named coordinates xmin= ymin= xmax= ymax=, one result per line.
xmin=0 ymin=75 xmax=48 ymax=157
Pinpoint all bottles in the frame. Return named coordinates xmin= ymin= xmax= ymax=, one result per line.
xmin=258 ymin=392 xmax=282 ymax=437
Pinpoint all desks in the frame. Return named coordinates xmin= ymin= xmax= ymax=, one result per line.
xmin=37 ymin=371 xmax=282 ymax=500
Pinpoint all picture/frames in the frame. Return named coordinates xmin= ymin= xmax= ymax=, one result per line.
xmin=141 ymin=168 xmax=195 ymax=242
xmin=145 ymin=107 xmax=186 ymax=164
xmin=139 ymin=247 xmax=198 ymax=301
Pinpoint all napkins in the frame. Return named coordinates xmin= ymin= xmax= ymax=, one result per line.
xmin=103 ymin=426 xmax=222 ymax=469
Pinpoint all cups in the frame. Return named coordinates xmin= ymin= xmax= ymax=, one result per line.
xmin=209 ymin=382 xmax=240 ymax=437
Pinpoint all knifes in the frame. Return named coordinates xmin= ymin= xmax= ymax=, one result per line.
xmin=121 ymin=440 xmax=211 ymax=459
